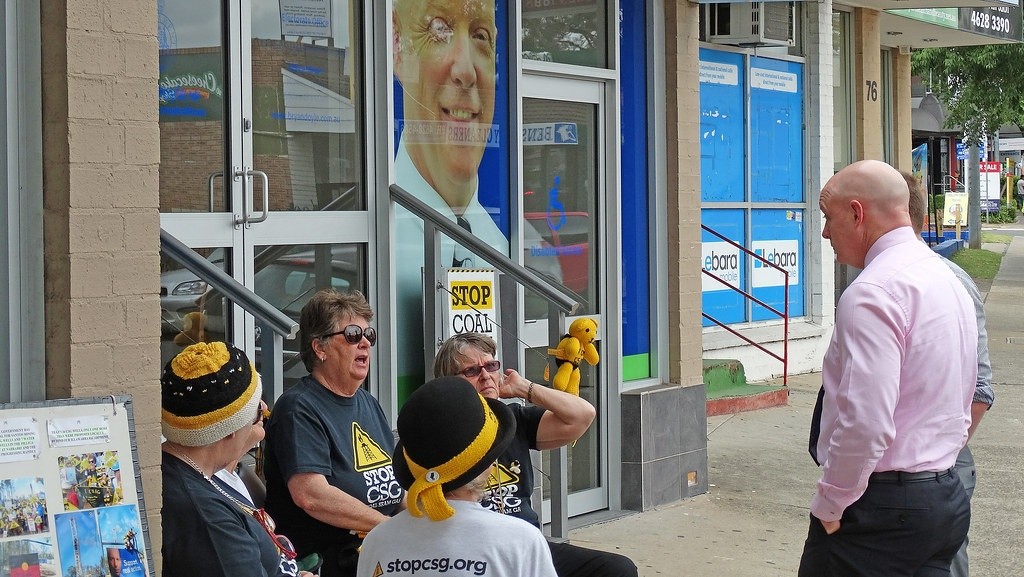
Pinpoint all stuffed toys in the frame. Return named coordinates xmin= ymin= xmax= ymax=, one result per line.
xmin=174 ymin=312 xmax=207 ymax=347
xmin=548 ymin=318 xmax=598 ymax=398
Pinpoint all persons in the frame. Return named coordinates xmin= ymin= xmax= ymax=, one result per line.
xmin=1017 ymin=175 xmax=1024 ymax=212
xmin=162 ymin=341 xmax=323 ymax=577
xmin=796 ymin=159 xmax=996 ymax=577
xmin=388 ymin=0 xmax=512 ymax=373
xmin=107 ymin=548 xmax=124 ymax=577
xmin=58 ymin=450 xmax=124 ymax=511
xmin=357 ymin=378 xmax=557 ymax=577
xmin=264 ymin=290 xmax=411 ymax=577
xmin=433 ymin=333 xmax=638 ymax=577
xmin=0 ymin=500 xmax=48 ymax=537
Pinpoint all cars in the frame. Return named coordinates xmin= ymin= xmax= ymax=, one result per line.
xmin=255 ymin=246 xmax=367 ymax=386
xmin=160 ymin=247 xmax=228 ymax=335
xmin=520 ymin=209 xmax=593 ymax=301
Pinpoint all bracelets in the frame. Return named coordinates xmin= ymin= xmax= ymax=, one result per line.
xmin=528 ymin=382 xmax=534 ymax=403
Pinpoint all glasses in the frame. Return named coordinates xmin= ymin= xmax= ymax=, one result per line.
xmin=453 ymin=360 xmax=501 ymax=378
xmin=88 ymin=460 xmax=95 ymax=464
xmin=252 ymin=403 xmax=262 ymax=426
xmin=322 ymin=324 xmax=377 ymax=346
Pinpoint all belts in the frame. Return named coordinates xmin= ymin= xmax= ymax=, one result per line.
xmin=868 ymin=465 xmax=957 ymax=486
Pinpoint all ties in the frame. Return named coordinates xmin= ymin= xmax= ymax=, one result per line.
xmin=808 ymin=382 xmax=827 ymax=468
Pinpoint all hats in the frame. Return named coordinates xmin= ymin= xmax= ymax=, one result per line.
xmin=161 ymin=341 xmax=263 ymax=447
xmin=392 ymin=375 xmax=516 ymax=520
